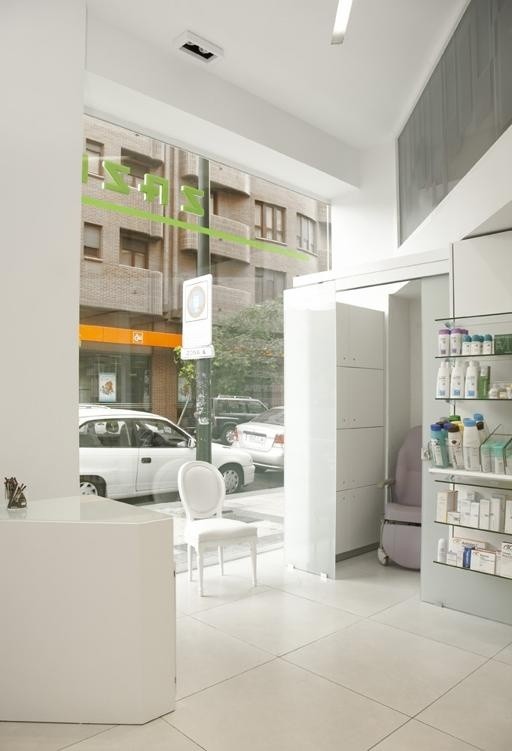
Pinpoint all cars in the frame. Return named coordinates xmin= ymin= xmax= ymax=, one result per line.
xmin=79 ymin=403 xmax=254 ymax=504
xmin=233 ymin=404 xmax=284 ymax=475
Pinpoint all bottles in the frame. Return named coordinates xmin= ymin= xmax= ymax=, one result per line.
xmin=429 ymin=413 xmax=488 ymax=472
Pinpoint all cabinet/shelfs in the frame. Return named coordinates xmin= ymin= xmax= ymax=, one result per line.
xmin=337 ymin=301 xmax=385 ymax=555
xmin=429 ymin=310 xmax=512 ymax=580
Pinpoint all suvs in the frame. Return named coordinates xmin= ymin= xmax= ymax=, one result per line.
xmin=193 ymin=392 xmax=274 ymax=445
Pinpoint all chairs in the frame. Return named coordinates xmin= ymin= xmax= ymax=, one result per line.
xmin=378 ymin=424 xmax=422 ymax=569
xmin=151 ymin=459 xmax=196 ymax=546
xmin=177 ymin=460 xmax=258 ymax=597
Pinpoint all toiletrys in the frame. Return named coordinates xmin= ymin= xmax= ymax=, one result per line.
xmin=429 ymin=327 xmax=512 ymax=581
